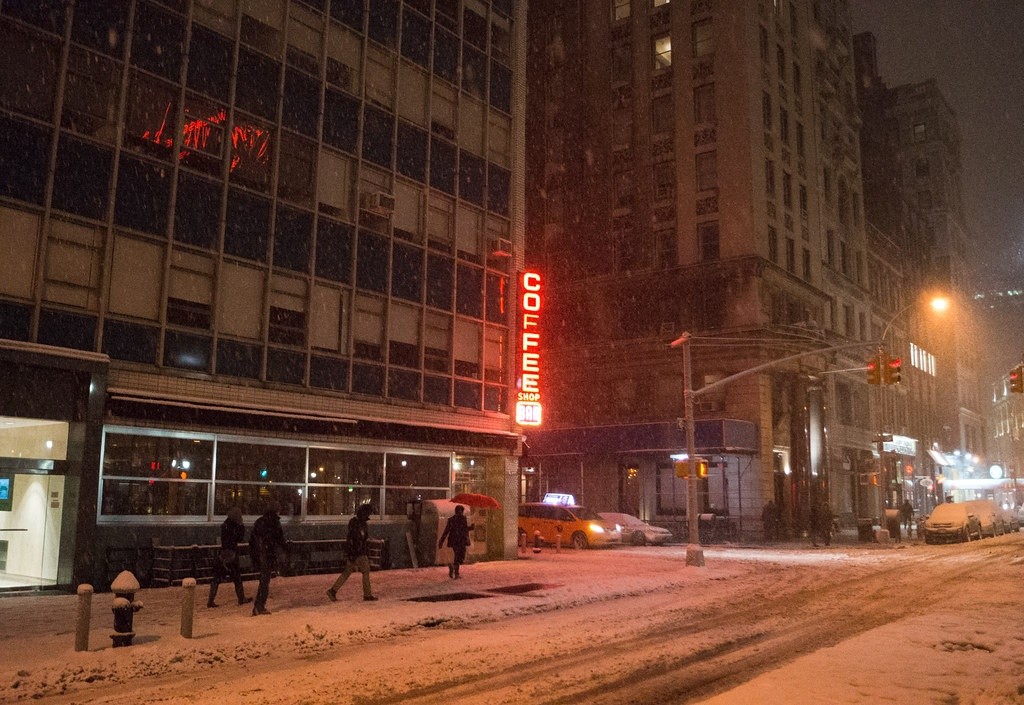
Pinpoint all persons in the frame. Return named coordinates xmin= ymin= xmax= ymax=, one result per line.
xmin=248 ymin=502 xmax=300 ymax=616
xmin=438 ymin=505 xmax=475 ymax=579
xmin=206 ymin=507 xmax=253 ymax=608
xmin=900 ymin=500 xmax=914 ymax=529
xmin=326 ymin=504 xmax=379 ymax=602
xmin=818 ymin=502 xmax=833 ymax=546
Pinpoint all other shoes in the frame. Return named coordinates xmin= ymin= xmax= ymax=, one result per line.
xmin=238 ymin=597 xmax=253 ymax=605
xmin=326 ymin=588 xmax=337 ymax=602
xmin=207 ymin=602 xmax=219 ymax=608
xmin=253 ymin=607 xmax=271 ymax=616
xmin=455 ymin=576 xmax=461 ymax=579
xmin=449 ymin=563 xmax=453 ymax=577
xmin=363 ymin=595 xmax=378 ymax=602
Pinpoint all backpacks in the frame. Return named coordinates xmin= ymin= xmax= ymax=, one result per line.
xmin=249 ymin=522 xmax=275 ymax=562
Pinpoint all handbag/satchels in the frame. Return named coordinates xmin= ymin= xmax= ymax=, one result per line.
xmin=217 ymin=548 xmax=248 ymax=571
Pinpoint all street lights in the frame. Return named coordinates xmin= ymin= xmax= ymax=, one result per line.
xmin=876 ymin=295 xmax=948 ymax=546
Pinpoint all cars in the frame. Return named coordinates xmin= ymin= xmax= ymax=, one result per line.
xmin=921 ymin=500 xmax=1024 ymax=544
xmin=518 ymin=493 xmax=621 ymax=549
xmin=599 ymin=513 xmax=673 ymax=546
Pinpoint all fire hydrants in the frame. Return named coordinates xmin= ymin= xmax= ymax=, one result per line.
xmin=109 ymin=571 xmax=144 ymax=649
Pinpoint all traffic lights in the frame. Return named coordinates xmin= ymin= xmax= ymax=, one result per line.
xmin=881 ymin=355 xmax=903 ymax=389
xmin=1010 ymin=362 xmax=1024 ymax=395
xmin=870 ymin=473 xmax=879 ymax=486
xmin=863 ymin=352 xmax=881 ymax=386
xmin=696 ymin=459 xmax=709 ymax=480
xmin=674 ymin=461 xmax=687 ymax=479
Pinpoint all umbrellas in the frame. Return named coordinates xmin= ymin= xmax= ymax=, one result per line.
xmin=448 ymin=493 xmax=501 ymax=510
